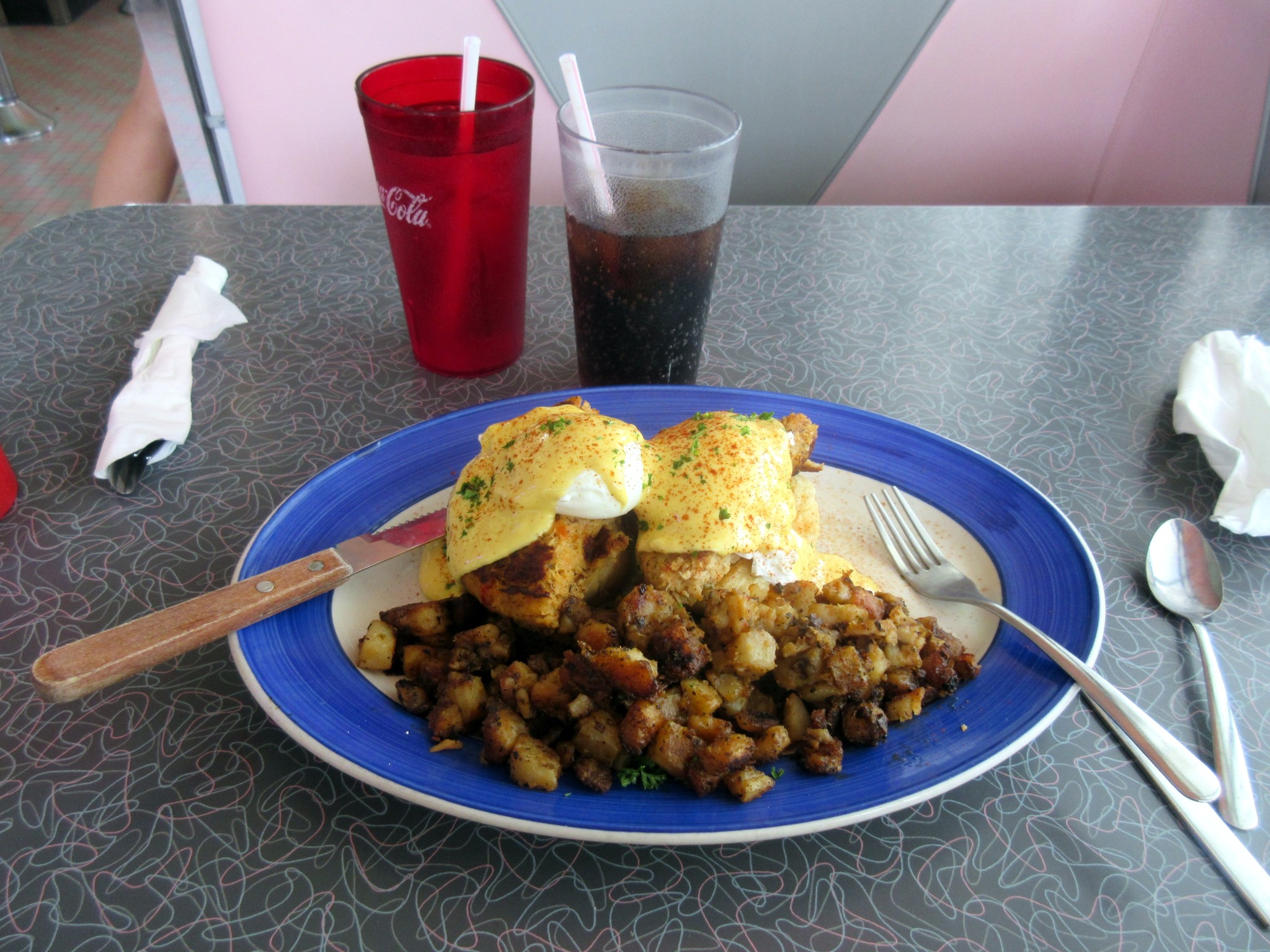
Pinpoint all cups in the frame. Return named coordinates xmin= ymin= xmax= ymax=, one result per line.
xmin=355 ymin=54 xmax=535 ymax=378
xmin=556 ymin=86 xmax=742 ymax=387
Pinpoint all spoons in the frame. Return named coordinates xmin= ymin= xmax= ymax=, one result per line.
xmin=1144 ymin=517 xmax=1259 ymax=832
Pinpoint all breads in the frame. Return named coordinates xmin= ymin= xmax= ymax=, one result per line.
xmin=441 ymin=404 xmax=876 ymax=627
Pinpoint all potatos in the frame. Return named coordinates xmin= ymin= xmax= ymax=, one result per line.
xmin=358 ymin=573 xmax=983 ymax=802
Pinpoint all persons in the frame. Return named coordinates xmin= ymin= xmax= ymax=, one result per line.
xmin=89 ymin=51 xmax=179 ymax=211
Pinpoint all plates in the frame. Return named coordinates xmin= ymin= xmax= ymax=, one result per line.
xmin=227 ymin=385 xmax=1109 ymax=843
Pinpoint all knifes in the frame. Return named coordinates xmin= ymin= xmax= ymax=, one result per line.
xmin=31 ymin=506 xmax=446 ymax=705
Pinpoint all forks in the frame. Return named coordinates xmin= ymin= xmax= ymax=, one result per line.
xmin=862 ymin=485 xmax=1225 ymax=802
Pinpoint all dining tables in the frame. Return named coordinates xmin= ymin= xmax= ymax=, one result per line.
xmin=2 ymin=202 xmax=1268 ymax=952
